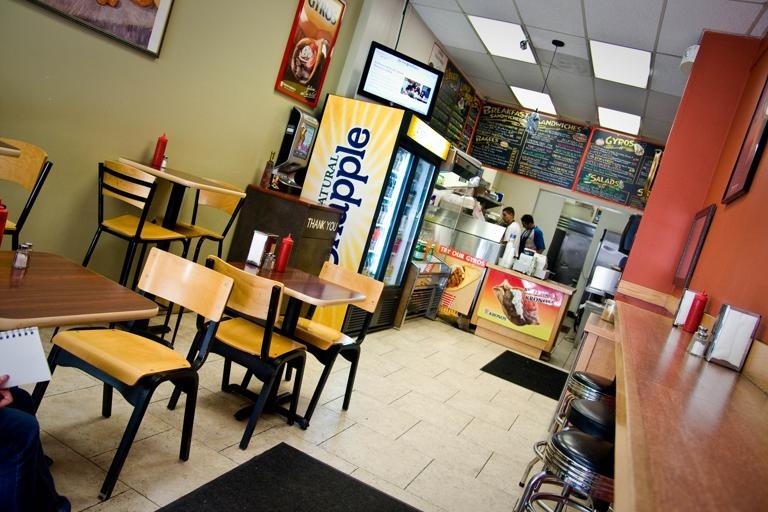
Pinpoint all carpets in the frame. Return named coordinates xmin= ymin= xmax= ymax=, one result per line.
xmin=154 ymin=442 xmax=424 ymax=512
xmin=480 ymin=350 xmax=568 ymax=402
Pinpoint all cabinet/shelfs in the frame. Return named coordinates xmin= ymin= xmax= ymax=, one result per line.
xmin=548 ymin=313 xmax=616 ymax=432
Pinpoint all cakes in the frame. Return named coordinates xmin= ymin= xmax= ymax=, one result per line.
xmin=491 ymin=279 xmax=540 ymax=327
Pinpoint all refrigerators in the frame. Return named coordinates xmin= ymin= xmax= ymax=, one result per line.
xmin=299 ymin=93 xmax=452 ymax=339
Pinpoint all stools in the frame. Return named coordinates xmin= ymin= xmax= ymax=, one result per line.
xmin=512 ymin=371 xmax=614 ymax=512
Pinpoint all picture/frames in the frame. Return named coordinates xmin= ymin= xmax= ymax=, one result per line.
xmin=274 ymin=0 xmax=347 ymax=108
xmin=721 ymin=75 xmax=768 ymax=204
xmin=672 ymin=203 xmax=716 ymax=289
xmin=29 ymin=0 xmax=174 ymax=58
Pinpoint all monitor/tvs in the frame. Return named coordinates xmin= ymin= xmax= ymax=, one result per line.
xmin=357 ymin=41 xmax=445 ymax=120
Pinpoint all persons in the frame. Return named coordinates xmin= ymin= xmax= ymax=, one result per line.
xmin=0 ymin=372 xmax=72 ymax=512
xmin=518 ymin=214 xmax=546 ymax=258
xmin=502 ymin=207 xmax=523 ymax=261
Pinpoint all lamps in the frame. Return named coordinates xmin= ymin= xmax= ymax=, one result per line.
xmin=525 ymin=38 xmax=565 ymax=135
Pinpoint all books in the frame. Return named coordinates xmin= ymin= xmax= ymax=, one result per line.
xmin=0 ymin=326 xmax=53 ymax=389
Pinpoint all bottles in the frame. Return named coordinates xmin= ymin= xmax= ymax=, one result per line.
xmin=682 ymin=287 xmax=708 ymax=357
xmin=152 ymin=132 xmax=168 ymax=168
xmin=260 ymin=151 xmax=275 ymax=190
xmin=262 ymin=233 xmax=295 ymax=273
xmin=12 ymin=242 xmax=33 ymax=268
xmin=413 ymin=239 xmax=436 ymax=261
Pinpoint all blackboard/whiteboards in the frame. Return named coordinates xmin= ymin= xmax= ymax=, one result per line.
xmin=426 ymin=56 xmax=666 ymax=210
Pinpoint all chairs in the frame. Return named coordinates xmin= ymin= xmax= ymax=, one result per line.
xmin=0 ymin=135 xmax=385 ymax=502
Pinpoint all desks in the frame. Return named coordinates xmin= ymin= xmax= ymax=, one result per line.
xmin=612 ymin=300 xmax=768 ymax=512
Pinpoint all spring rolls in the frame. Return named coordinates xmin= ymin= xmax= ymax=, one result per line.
xmin=444 ymin=264 xmax=483 ymax=291
